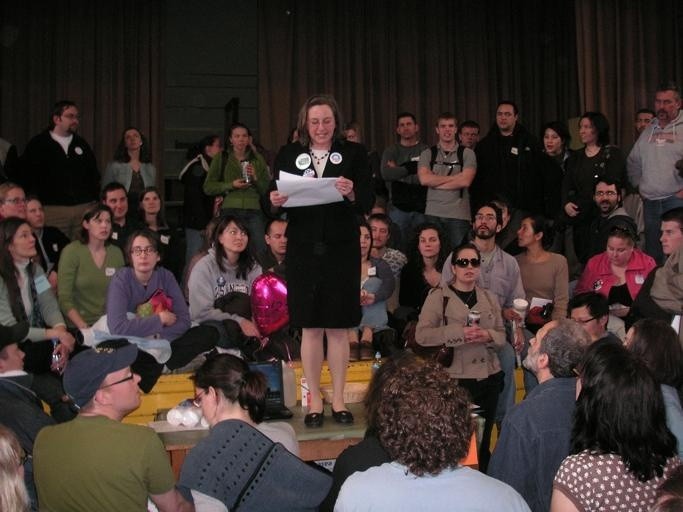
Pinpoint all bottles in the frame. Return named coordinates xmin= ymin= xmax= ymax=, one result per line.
xmin=240 ymin=160 xmax=252 ymax=184
xmin=371 ymin=350 xmax=382 ymax=370
xmin=50 ymin=337 xmax=62 ymax=372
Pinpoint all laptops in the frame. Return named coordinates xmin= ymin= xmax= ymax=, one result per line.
xmin=246 ymin=361 xmax=293 ymax=421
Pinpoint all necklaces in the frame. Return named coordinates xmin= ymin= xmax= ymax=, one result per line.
xmin=310 ymin=148 xmax=330 ymax=165
xmin=453 ymin=282 xmax=475 ymax=309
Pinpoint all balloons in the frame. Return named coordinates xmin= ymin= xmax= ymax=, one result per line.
xmin=251 ymin=272 xmax=289 ymax=336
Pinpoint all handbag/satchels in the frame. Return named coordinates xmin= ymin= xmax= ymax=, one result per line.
xmin=524 ymin=302 xmax=554 ymax=334
xmin=244 ymin=343 xmax=293 ymax=419
xmin=408 ymin=295 xmax=453 ymax=368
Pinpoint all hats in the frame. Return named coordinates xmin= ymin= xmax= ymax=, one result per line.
xmin=63 ymin=343 xmax=138 ymax=409
xmin=0 ymin=317 xmax=30 ymax=349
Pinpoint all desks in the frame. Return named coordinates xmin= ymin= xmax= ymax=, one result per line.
xmin=155 ymin=402 xmax=485 ymax=512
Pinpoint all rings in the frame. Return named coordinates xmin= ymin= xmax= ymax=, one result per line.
xmin=342 ymin=187 xmax=346 ymax=191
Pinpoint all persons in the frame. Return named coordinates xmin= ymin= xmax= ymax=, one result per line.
xmin=332 ymin=359 xmax=531 ymax=509
xmin=257 ymin=95 xmax=377 ymax=433
xmin=0 ymin=427 xmax=31 ymax=511
xmin=550 ymin=337 xmax=681 ymax=512
xmin=177 ymin=353 xmax=301 ymax=511
xmin=0 ymin=82 xmax=681 ymax=431
xmin=33 ymin=345 xmax=187 ymax=510
xmin=488 ymin=319 xmax=591 ymax=512
xmin=0 ymin=319 xmax=61 ymax=511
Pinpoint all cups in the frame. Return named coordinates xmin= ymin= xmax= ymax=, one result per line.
xmin=164 ymin=400 xmax=210 ymax=429
xmin=466 ymin=310 xmax=481 ymax=327
xmin=512 ymin=297 xmax=528 ymax=318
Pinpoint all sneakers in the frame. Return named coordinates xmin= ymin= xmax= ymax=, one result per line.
xmin=349 ymin=341 xmax=361 ymax=361
xmin=359 ymin=340 xmax=374 ymax=361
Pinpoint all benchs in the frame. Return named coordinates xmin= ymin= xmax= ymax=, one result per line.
xmin=112 ymin=372 xmax=196 ymax=426
xmin=293 ymin=359 xmax=387 ymax=408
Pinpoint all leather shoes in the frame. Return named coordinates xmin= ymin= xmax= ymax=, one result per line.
xmin=304 ymin=409 xmax=324 ymax=429
xmin=332 ymin=407 xmax=354 ymax=423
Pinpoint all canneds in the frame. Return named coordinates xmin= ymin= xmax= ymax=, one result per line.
xmin=466 ymin=310 xmax=481 ymax=327
xmin=239 ymin=159 xmax=251 ymax=184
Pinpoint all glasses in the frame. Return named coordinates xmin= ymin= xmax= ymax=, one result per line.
xmin=5 ymin=198 xmax=30 ymax=205
xmin=192 ymin=392 xmax=205 ymax=407
xmin=20 ymin=446 xmax=29 ymax=467
xmin=454 ymin=257 xmax=480 ymax=268
xmin=100 ymin=370 xmax=134 ymax=390
xmin=131 ymin=246 xmax=157 ymax=256
xmin=596 ymin=192 xmax=617 ymax=196
xmin=576 ymin=318 xmax=593 ymax=325
